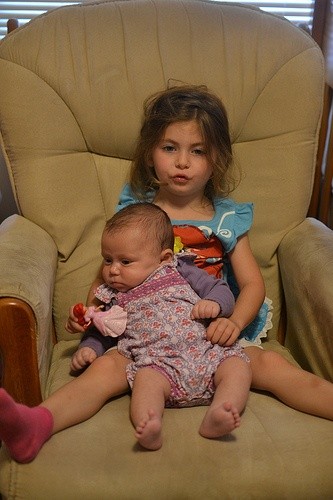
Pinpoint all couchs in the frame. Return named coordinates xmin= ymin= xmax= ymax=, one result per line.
xmin=0 ymin=0 xmax=333 ymax=500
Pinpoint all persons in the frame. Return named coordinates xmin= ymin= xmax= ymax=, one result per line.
xmin=0 ymin=79 xmax=332 ymax=466
xmin=71 ymin=303 xmax=129 ymax=337
xmin=64 ymin=201 xmax=254 ymax=452
